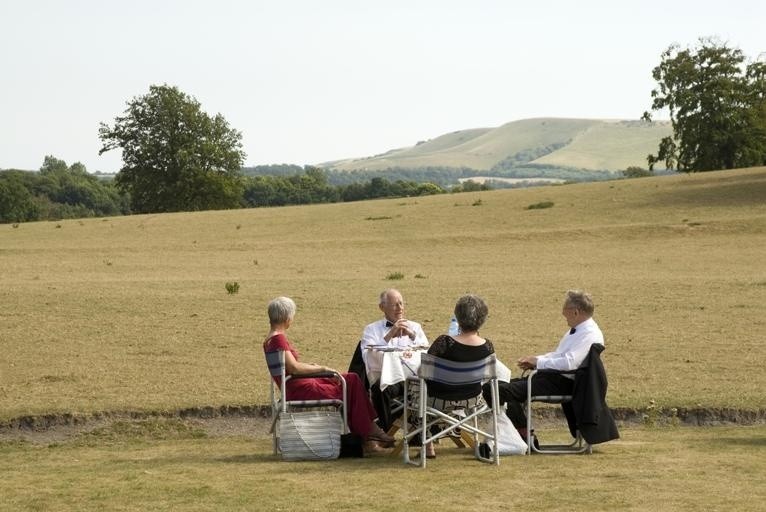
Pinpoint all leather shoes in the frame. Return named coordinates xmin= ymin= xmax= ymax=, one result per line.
xmin=527 ymin=439 xmax=540 ymax=452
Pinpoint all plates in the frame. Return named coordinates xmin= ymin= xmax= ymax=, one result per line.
xmin=365 ymin=343 xmax=396 ymax=352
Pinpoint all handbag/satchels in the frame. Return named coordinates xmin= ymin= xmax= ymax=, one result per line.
xmin=479 ymin=442 xmax=491 ymax=459
xmin=279 ymin=411 xmax=341 ymax=462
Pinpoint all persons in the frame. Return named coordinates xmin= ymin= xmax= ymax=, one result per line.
xmin=361 ymin=289 xmax=496 ymax=459
xmin=263 ymin=297 xmax=396 ymax=457
xmin=482 ymin=291 xmax=605 ymax=454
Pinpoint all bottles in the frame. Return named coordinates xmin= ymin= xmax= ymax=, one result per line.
xmin=449 ymin=318 xmax=459 ymax=337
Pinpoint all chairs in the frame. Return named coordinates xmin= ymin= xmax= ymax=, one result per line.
xmin=519 ymin=342 xmax=606 ymax=455
xmin=402 ymin=351 xmax=504 ymax=469
xmin=261 ymin=348 xmax=350 ymax=456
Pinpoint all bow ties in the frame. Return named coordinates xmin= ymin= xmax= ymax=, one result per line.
xmin=386 ymin=321 xmax=393 ymax=327
xmin=569 ymin=328 xmax=576 ymax=335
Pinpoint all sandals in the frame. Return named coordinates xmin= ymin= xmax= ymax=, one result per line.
xmin=362 ymin=428 xmax=394 ymax=457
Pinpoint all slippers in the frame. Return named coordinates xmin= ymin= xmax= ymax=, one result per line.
xmin=414 ymin=452 xmax=436 ymax=459
